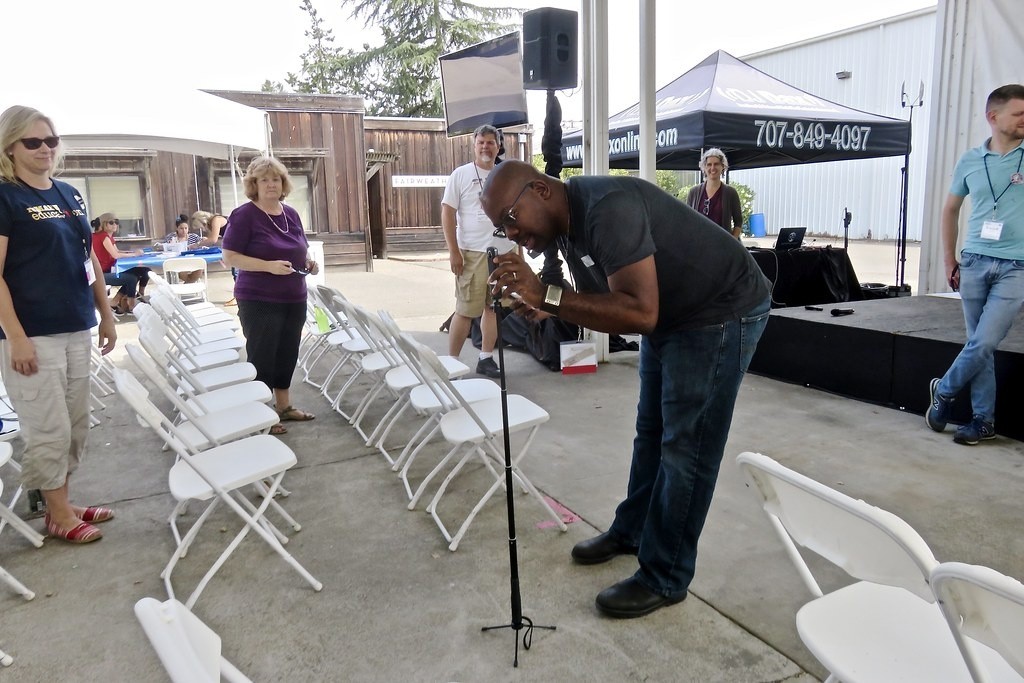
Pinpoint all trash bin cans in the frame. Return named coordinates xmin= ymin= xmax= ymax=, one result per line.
xmin=860 ymin=282 xmax=889 ymax=298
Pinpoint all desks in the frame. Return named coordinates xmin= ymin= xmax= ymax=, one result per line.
xmin=749 ymin=246 xmax=865 ymax=308
xmin=115 ymin=248 xmax=222 ymax=278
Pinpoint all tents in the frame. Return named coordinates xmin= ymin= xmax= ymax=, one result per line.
xmin=559 ymin=49 xmax=912 ymax=290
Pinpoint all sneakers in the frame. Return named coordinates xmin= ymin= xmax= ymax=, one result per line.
xmin=112 ymin=305 xmax=124 ymax=316
xmin=476 ymin=357 xmax=500 ymax=378
xmin=925 ymin=378 xmax=955 ymax=431
xmin=954 ymin=414 xmax=996 ymax=444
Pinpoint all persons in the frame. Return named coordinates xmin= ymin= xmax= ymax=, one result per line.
xmin=684 ymin=148 xmax=742 ymax=241
xmin=439 ymin=125 xmax=517 ymax=379
xmin=164 ymin=214 xmax=203 ymax=283
xmin=222 ymin=155 xmax=320 ymax=435
xmin=186 ymin=210 xmax=240 ymax=306
xmin=924 ymin=84 xmax=1024 ymax=446
xmin=482 ymin=160 xmax=773 ymax=620
xmin=90 ymin=212 xmax=152 ymax=316
xmin=1 ymin=105 xmax=118 ymax=544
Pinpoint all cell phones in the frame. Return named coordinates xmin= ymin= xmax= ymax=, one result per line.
xmin=488 ymin=247 xmax=504 ymax=299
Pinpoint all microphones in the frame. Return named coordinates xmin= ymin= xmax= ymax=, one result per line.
xmin=831 ymin=308 xmax=855 ymax=316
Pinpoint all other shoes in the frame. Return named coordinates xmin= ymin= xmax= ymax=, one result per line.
xmin=48 ymin=516 xmax=102 ymax=543
xmin=225 ymin=297 xmax=237 ymax=306
xmin=45 ymin=506 xmax=114 ymax=522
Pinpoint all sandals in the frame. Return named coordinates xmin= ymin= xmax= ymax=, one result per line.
xmin=270 ymin=423 xmax=287 ymax=434
xmin=273 ymin=403 xmax=315 ymax=422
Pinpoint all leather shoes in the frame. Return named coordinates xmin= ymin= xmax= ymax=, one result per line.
xmin=571 ymin=530 xmax=643 ymax=562
xmin=596 ymin=574 xmax=687 ymax=615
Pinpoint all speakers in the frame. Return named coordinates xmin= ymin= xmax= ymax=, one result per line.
xmin=522 ymin=7 xmax=579 ymax=90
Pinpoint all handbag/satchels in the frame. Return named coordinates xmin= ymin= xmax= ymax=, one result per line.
xmin=559 ymin=340 xmax=598 ymax=374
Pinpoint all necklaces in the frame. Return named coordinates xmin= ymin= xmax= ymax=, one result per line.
xmin=251 ymin=198 xmax=289 ymax=234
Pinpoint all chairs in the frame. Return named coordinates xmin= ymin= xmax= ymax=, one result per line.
xmin=736 ymin=452 xmax=1024 ymax=682
xmin=112 ymin=257 xmax=322 ymax=683
xmin=297 ymin=285 xmax=567 ymax=551
xmin=929 ymin=562 xmax=1024 ymax=682
xmin=0 ymin=285 xmax=124 ymax=667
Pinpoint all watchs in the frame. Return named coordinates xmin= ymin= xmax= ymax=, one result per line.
xmin=538 ymin=277 xmax=564 ymax=314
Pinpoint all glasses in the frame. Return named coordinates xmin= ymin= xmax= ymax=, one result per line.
xmin=14 ymin=137 xmax=59 ymax=150
xmin=492 ymin=183 xmax=532 ymax=238
xmin=291 ymin=262 xmax=315 ymax=274
xmin=703 ymin=199 xmax=710 ymax=215
xmin=951 ymin=263 xmax=962 ymax=294
xmin=107 ymin=220 xmax=119 ymax=225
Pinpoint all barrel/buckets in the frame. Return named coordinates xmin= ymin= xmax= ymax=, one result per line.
xmin=750 ymin=212 xmax=767 ymax=237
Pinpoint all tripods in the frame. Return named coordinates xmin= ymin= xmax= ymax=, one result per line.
xmin=481 ymin=297 xmax=558 ymax=668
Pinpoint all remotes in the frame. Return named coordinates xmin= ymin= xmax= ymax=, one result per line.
xmin=806 ymin=306 xmax=824 ymax=311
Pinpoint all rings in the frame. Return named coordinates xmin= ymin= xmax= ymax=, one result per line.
xmin=512 ymin=271 xmax=517 ymax=282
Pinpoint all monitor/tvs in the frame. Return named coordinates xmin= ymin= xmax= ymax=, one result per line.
xmin=437 ymin=30 xmax=530 ymax=138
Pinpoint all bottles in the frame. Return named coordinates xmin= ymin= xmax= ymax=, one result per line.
xmin=313 ymin=303 xmax=330 ymax=333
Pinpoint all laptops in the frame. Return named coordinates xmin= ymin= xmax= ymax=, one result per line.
xmin=761 ymin=227 xmax=808 ymax=251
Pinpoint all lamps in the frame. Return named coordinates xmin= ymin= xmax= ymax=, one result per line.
xmin=836 ymin=72 xmax=850 ymax=79
